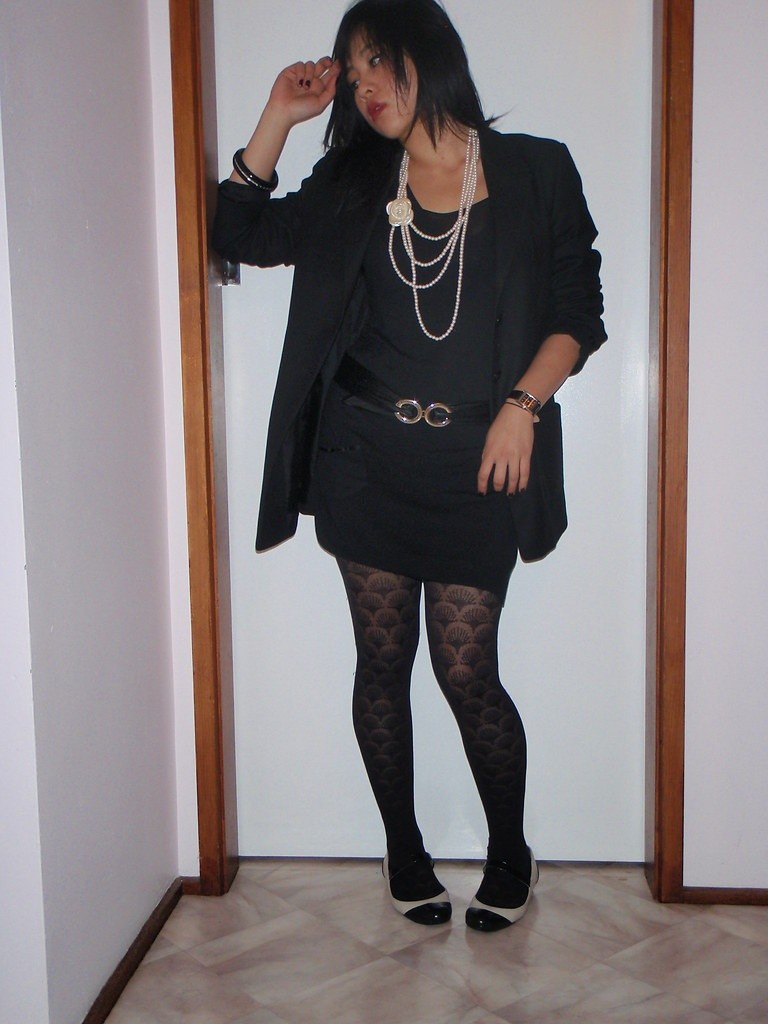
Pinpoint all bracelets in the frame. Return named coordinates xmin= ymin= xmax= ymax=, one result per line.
xmin=233 ymin=148 xmax=278 ymax=193
xmin=502 ymin=401 xmax=536 ymax=419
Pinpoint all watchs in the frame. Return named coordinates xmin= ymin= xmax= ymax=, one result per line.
xmin=505 ymin=391 xmax=545 ymax=423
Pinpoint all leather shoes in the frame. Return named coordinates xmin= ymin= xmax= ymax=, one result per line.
xmin=465 ymin=847 xmax=539 ymax=931
xmin=380 ymin=854 xmax=452 ymax=925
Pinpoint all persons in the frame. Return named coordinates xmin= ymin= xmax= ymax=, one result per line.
xmin=207 ymin=0 xmax=608 ymax=933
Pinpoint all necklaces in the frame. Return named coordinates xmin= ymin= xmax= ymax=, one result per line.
xmin=386 ymin=127 xmax=480 ymax=342
